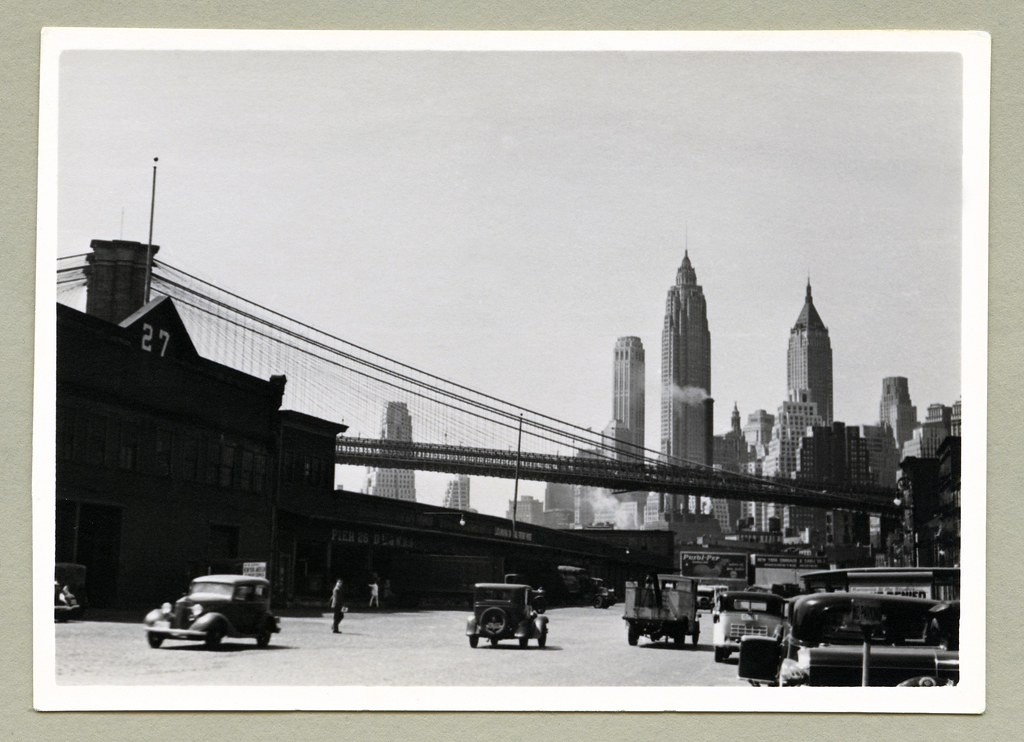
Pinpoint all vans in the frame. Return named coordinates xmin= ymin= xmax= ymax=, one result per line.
xmin=713 ymin=590 xmax=786 ymax=662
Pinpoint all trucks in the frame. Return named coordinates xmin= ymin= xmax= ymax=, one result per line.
xmin=397 ymin=552 xmax=547 ymax=613
xmin=556 ymin=565 xmax=618 ymax=609
xmin=622 ymin=574 xmax=701 ymax=651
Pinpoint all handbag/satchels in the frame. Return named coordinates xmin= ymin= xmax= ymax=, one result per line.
xmin=341 ymin=607 xmax=348 ymax=612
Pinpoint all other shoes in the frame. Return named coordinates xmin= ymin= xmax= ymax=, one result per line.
xmin=333 ymin=631 xmax=341 ymax=633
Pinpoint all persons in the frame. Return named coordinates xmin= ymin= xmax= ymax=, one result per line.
xmin=331 ymin=578 xmax=346 ymax=633
xmin=369 ymin=582 xmax=380 ymax=607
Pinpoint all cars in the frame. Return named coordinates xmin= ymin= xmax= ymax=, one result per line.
xmin=466 ymin=583 xmax=548 ymax=648
xmin=785 ymin=565 xmax=959 ymax=687
xmin=55 ymin=580 xmax=78 ymax=622
xmin=143 ymin=574 xmax=280 ymax=649
